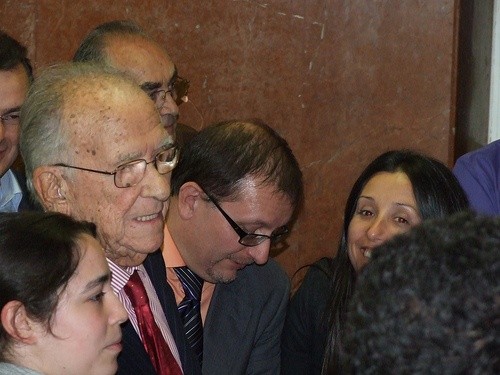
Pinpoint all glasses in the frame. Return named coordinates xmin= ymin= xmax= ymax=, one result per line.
xmin=147 ymin=76 xmax=190 ymax=110
xmin=190 ymin=180 xmax=290 ymax=246
xmin=48 ymin=142 xmax=180 ymax=188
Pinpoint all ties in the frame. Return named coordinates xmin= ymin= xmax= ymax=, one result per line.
xmin=124 ymin=269 xmax=184 ymax=375
xmin=177 ymin=265 xmax=205 ymax=369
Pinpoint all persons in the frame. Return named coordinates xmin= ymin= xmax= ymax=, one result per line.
xmin=0 ymin=21 xmax=500 ymax=374
